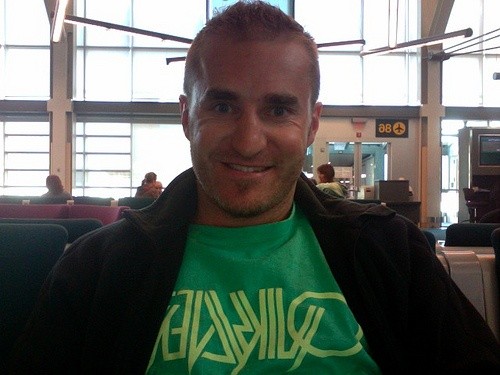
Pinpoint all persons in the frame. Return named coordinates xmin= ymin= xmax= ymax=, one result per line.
xmin=135 ymin=172 xmax=163 ymax=201
xmin=18 ymin=0 xmax=500 ymax=375
xmin=39 ymin=174 xmax=73 ymax=204
xmin=315 ymin=164 xmax=346 ymax=199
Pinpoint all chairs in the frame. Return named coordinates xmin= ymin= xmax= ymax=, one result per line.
xmin=0 ymin=187 xmax=500 ymax=375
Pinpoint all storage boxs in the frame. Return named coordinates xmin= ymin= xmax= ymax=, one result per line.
xmin=374 ymin=180 xmax=409 ymax=202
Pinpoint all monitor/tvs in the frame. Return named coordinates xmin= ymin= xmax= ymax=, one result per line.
xmin=471 ymin=128 xmax=500 ymax=176
